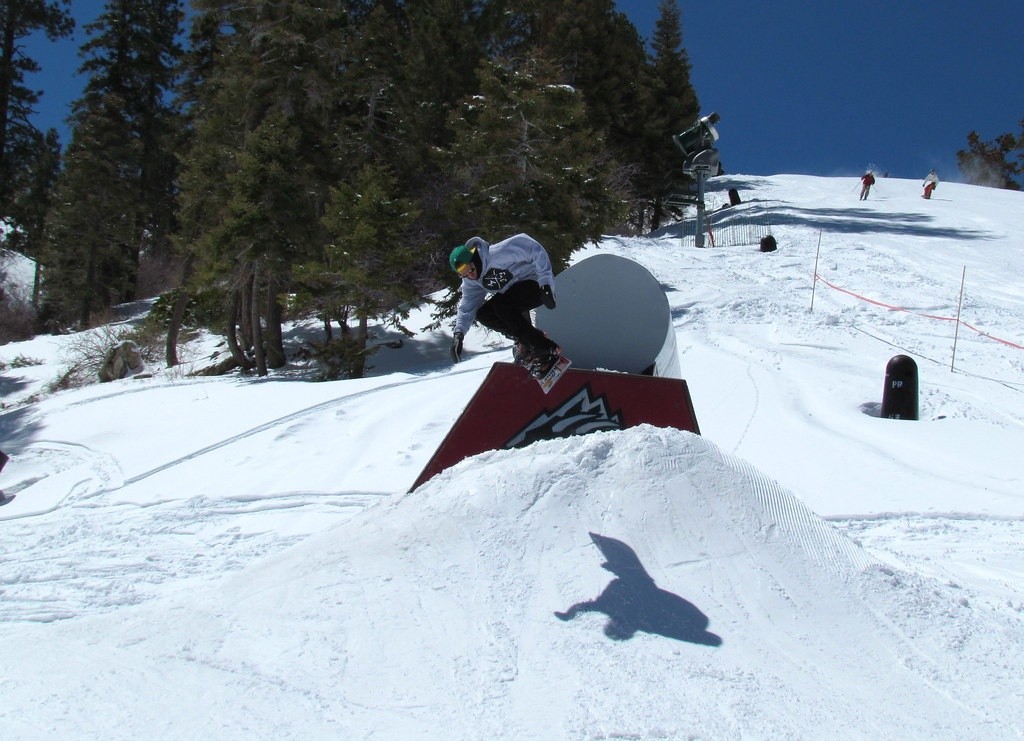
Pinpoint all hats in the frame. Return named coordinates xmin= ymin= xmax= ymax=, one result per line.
xmin=449 ymin=245 xmax=476 ymax=272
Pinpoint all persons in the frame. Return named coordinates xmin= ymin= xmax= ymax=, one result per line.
xmin=450 ymin=233 xmax=561 ymax=381
xmin=859 ymin=171 xmax=876 ymax=201
xmin=921 ymin=168 xmax=939 ymax=199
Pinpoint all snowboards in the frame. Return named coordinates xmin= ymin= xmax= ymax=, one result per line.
xmin=511 ymin=341 xmax=572 ymax=395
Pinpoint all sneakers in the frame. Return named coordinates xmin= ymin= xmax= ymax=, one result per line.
xmin=530 ymin=339 xmax=561 ymax=377
xmin=515 ymin=327 xmax=546 ymax=365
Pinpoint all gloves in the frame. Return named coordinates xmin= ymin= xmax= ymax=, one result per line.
xmin=449 ymin=332 xmax=464 ymax=364
xmin=540 ymin=285 xmax=556 ymax=309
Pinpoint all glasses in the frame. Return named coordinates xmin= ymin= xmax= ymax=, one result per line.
xmin=459 ymin=262 xmax=476 ymax=278
